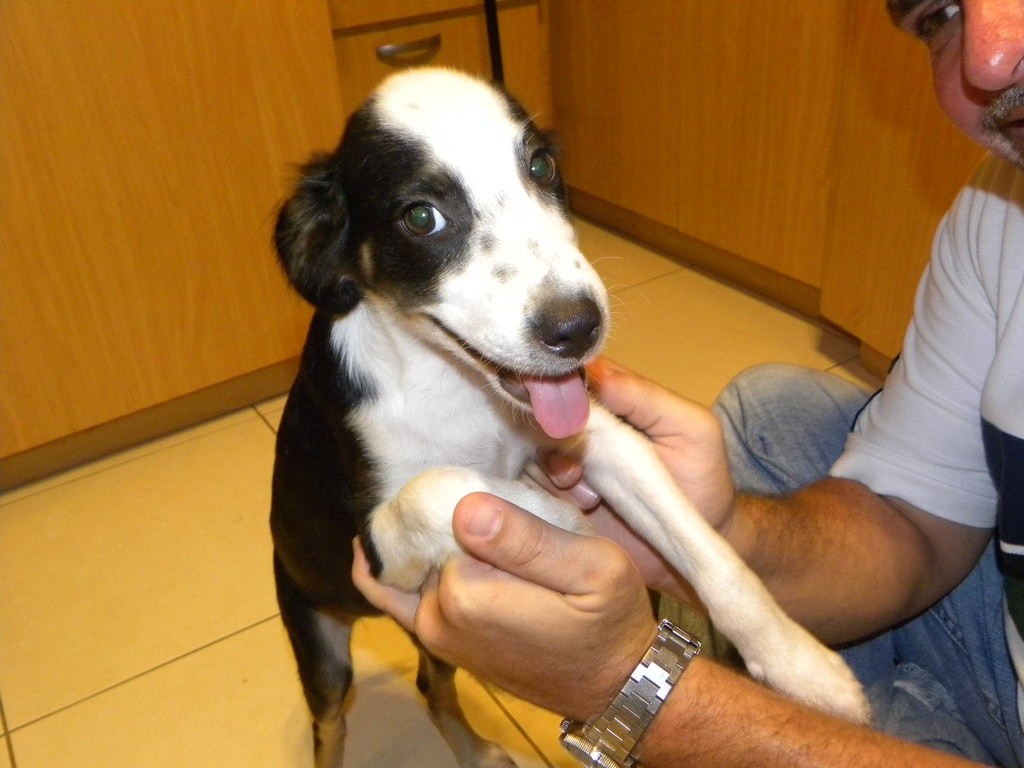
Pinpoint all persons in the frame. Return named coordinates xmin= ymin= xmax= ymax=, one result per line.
xmin=348 ymin=0 xmax=1024 ymax=768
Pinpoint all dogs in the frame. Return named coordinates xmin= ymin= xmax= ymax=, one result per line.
xmin=269 ymin=60 xmax=883 ymax=767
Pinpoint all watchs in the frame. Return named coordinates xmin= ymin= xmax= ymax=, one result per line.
xmin=557 ymin=617 xmax=701 ymax=768
